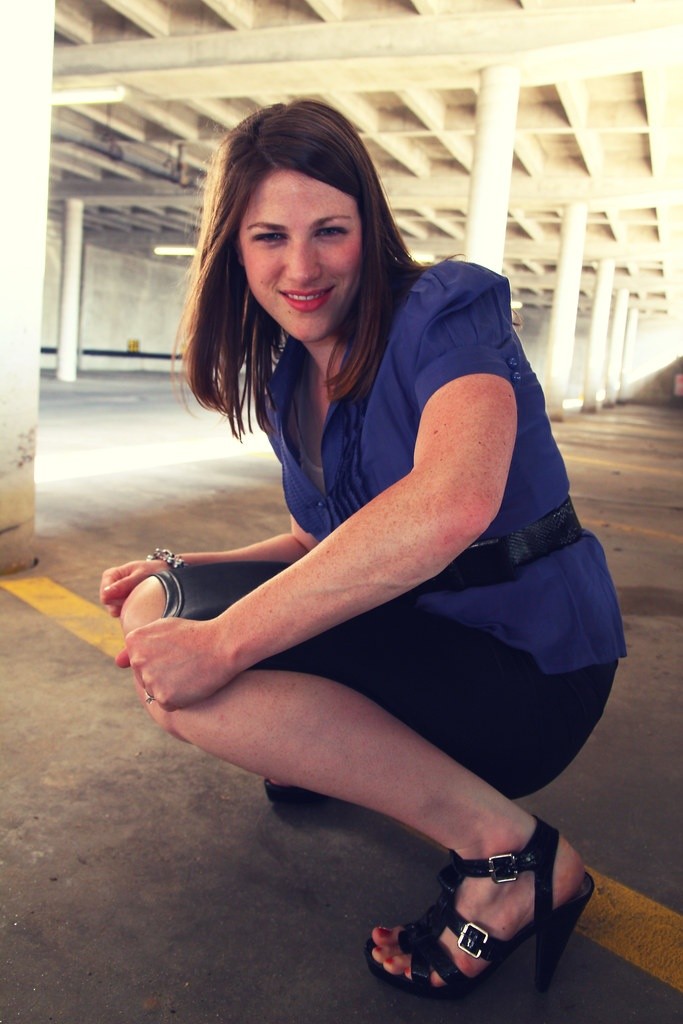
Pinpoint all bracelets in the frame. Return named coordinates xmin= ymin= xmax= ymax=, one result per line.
xmin=144 ymin=548 xmax=186 ymax=570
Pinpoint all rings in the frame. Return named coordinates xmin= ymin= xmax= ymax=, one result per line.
xmin=144 ymin=690 xmax=155 ymax=705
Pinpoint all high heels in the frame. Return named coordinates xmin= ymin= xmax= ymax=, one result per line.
xmin=264 ymin=779 xmax=329 ymax=806
xmin=363 ymin=815 xmax=595 ymax=995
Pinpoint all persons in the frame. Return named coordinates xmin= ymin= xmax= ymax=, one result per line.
xmin=99 ymin=100 xmax=630 ymax=1000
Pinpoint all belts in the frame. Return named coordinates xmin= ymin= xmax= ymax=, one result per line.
xmin=428 ymin=494 xmax=583 ymax=585
